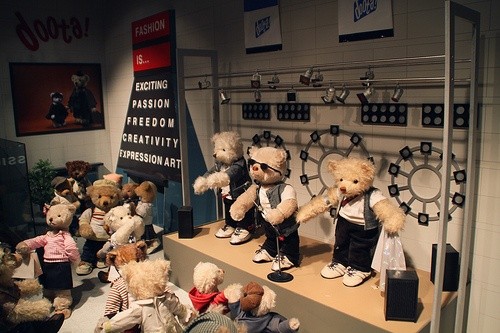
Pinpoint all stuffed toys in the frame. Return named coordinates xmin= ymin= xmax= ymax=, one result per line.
xmin=295 ymin=157 xmax=406 ymax=286
xmin=0 ymin=160 xmax=300 ymax=333
xmin=47 ymin=74 xmax=99 ymax=129
xmin=193 ymin=131 xmax=259 ymax=244
xmin=230 ymin=145 xmax=301 ymax=271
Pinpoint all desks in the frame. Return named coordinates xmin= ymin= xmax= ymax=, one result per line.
xmin=160 ymin=220 xmax=469 ymax=333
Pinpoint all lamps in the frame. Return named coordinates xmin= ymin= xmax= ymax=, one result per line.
xmin=197 ymin=71 xmax=404 ymax=104
